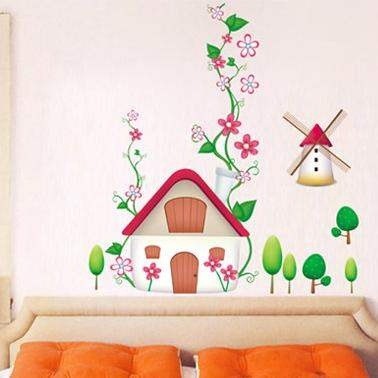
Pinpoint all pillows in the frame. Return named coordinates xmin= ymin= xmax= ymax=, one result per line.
xmin=6 ymin=340 xmax=185 ymax=378
xmin=194 ymin=344 xmax=369 ymax=378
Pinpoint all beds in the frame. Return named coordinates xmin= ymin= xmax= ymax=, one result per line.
xmin=0 ymin=296 xmax=378 ymax=378
